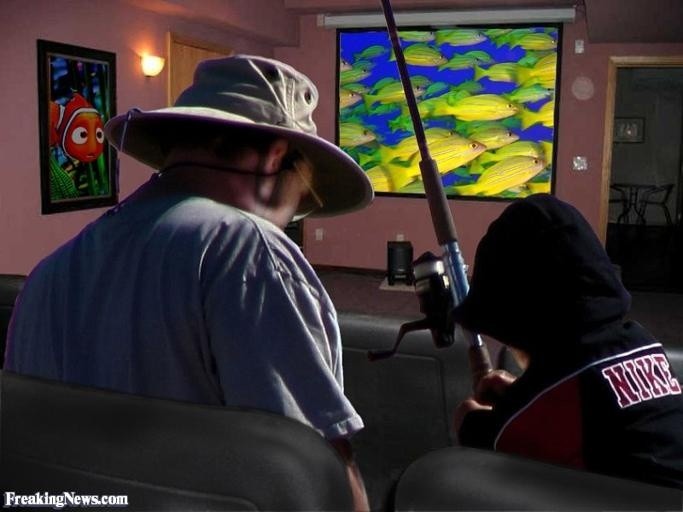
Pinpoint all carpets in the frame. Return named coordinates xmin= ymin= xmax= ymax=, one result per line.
xmin=378 ymin=268 xmax=450 ymax=295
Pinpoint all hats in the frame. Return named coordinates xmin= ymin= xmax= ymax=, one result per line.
xmin=104 ymin=54 xmax=376 ymax=220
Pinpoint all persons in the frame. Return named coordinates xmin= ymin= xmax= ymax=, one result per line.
xmin=0 ymin=54 xmax=375 ymax=512
xmin=455 ymin=193 xmax=683 ymax=490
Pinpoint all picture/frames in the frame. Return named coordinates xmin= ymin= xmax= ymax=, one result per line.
xmin=163 ymin=28 xmax=237 ymax=109
xmin=613 ymin=115 xmax=648 ymax=145
xmin=32 ymin=37 xmax=120 ymax=216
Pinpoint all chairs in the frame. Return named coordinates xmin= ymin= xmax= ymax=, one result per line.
xmin=496 ymin=340 xmax=682 ymax=394
xmin=1 ymin=272 xmax=29 ymax=355
xmin=326 ymin=306 xmax=484 ymax=452
xmin=0 ymin=365 xmax=360 ymax=511
xmin=602 ymin=185 xmax=631 ymax=225
xmin=388 ymin=443 xmax=682 ymax=509
xmin=636 ymin=181 xmax=677 ymax=225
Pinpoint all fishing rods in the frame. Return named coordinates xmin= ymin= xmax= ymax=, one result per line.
xmin=366 ymin=0 xmax=495 ymax=390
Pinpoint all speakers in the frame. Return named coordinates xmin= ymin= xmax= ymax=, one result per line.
xmin=387 ymin=241 xmax=413 ymax=287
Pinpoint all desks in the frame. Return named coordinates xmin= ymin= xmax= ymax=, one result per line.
xmin=609 ymin=182 xmax=655 ymax=227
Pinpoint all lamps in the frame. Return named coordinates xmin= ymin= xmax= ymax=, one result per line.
xmin=136 ymin=58 xmax=166 ymax=79
